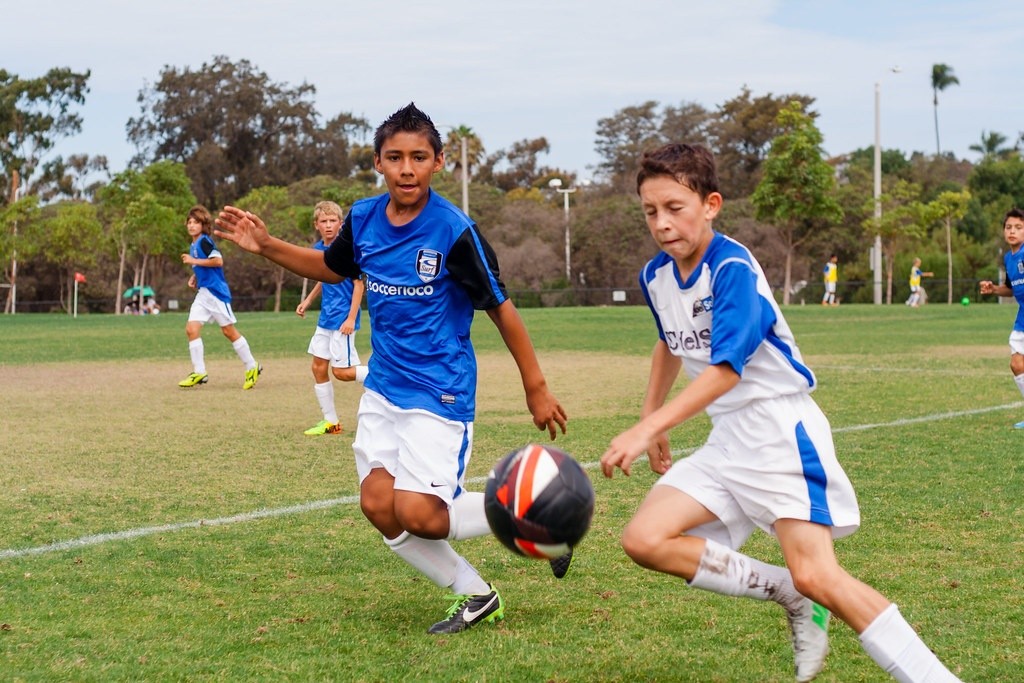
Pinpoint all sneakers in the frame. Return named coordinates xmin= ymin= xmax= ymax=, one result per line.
xmin=179 ymin=373 xmax=208 ymax=387
xmin=428 ymin=580 xmax=505 ymax=635
xmin=244 ymin=362 xmax=264 ymax=389
xmin=549 ymin=548 xmax=576 ymax=578
xmin=787 ymin=597 xmax=832 ymax=683
xmin=304 ymin=420 xmax=343 ymax=435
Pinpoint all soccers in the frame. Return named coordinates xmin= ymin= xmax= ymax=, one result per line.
xmin=485 ymin=441 xmax=594 ymax=559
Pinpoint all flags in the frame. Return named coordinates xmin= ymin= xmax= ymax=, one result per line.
xmin=74 ymin=272 xmax=86 ymax=282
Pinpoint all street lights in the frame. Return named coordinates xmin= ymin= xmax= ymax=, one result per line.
xmin=548 ymin=178 xmax=581 ymax=282
xmin=872 ymin=65 xmax=905 ymax=306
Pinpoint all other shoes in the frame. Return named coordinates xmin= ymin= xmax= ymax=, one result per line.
xmin=822 ymin=301 xmax=827 ymax=306
xmin=830 ymin=302 xmax=836 ymax=307
xmin=1013 ymin=421 xmax=1024 ymax=430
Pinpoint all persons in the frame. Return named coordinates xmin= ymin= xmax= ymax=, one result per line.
xmin=598 ymin=143 xmax=961 ymax=683
xmin=905 ymin=256 xmax=934 ymax=306
xmin=297 ymin=200 xmax=371 ymax=435
xmin=177 ymin=204 xmax=264 ymax=390
xmin=978 ymin=206 xmax=1024 ymax=429
xmin=124 ymin=295 xmax=161 ymax=314
xmin=214 ymin=101 xmax=570 ymax=634
xmin=823 ymin=254 xmax=839 ymax=307
